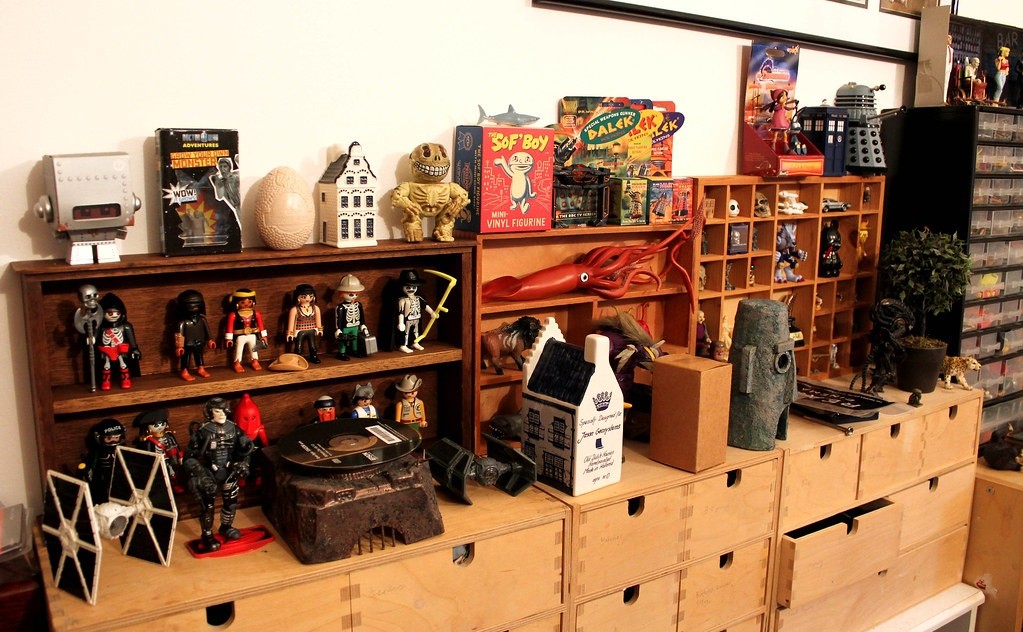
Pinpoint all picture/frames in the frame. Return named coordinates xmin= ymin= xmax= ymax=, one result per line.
xmin=531 ymin=0 xmax=960 ymax=65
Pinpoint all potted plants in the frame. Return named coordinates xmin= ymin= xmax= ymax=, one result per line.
xmin=880 ymin=227 xmax=972 ymax=393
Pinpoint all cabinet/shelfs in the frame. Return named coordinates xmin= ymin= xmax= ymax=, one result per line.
xmin=30 ymin=474 xmax=571 ymax=632
xmin=13 ymin=237 xmax=481 ymax=541
xmin=881 ymin=105 xmax=1023 ymax=431
xmin=458 ymin=221 xmax=693 ymax=463
xmin=532 ymin=436 xmax=782 ymax=632
xmin=780 ymin=370 xmax=987 ymax=632
xmin=688 ymin=174 xmax=889 ymax=384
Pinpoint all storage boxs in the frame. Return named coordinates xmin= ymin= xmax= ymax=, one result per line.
xmin=654 ymin=350 xmax=732 ymax=471
xmin=671 ymin=175 xmax=693 ymax=223
xmin=738 ymin=119 xmax=824 ymax=179
xmin=153 ymin=125 xmax=243 ymax=255
xmin=454 ymin=124 xmax=553 ymax=234
xmin=609 ymin=175 xmax=647 ymax=224
xmin=638 ymin=175 xmax=672 ymax=224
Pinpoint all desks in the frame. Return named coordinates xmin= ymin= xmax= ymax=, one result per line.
xmin=867 ymin=582 xmax=986 ymax=632
